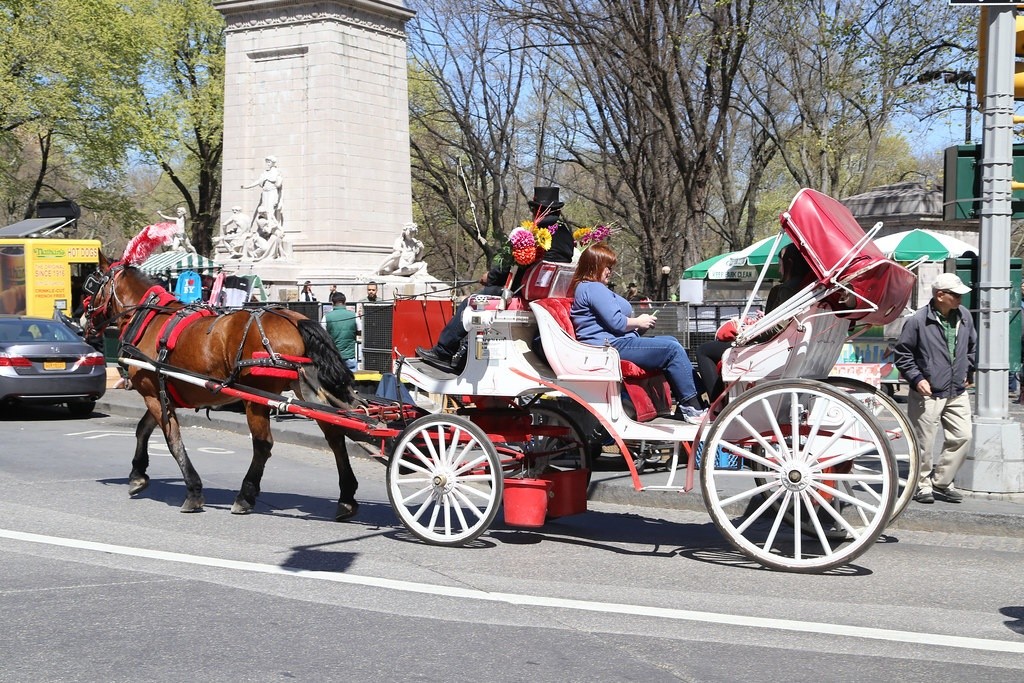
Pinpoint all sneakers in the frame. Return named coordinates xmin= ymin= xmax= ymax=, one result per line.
xmin=675 ymin=401 xmax=703 ymax=421
xmin=685 ymin=407 xmax=717 ymax=424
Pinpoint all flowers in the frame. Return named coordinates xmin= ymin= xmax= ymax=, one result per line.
xmin=573 ymin=224 xmax=611 ymax=246
xmin=509 ymin=212 xmax=558 ymax=265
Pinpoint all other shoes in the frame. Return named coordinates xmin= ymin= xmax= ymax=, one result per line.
xmin=932 ymin=483 xmax=963 ymax=499
xmin=912 ymin=493 xmax=934 ymax=503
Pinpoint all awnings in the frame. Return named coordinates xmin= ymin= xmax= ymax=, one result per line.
xmin=131 ymin=251 xmax=224 ymax=294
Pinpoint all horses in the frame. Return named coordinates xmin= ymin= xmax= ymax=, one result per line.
xmin=86 ymin=247 xmax=359 ymax=521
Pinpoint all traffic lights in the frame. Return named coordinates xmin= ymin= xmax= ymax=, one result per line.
xmin=976 ymin=4 xmax=1024 ymax=106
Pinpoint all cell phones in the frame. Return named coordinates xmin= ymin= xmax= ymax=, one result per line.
xmin=652 ymin=310 xmax=660 ymax=316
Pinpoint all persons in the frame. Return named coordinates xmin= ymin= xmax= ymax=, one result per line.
xmin=570 ymin=244 xmax=709 ymax=421
xmin=895 ymin=272 xmax=976 ymax=504
xmin=222 ymin=206 xmax=252 ymax=259
xmin=71 ymin=275 xmax=85 ymax=310
xmin=329 ymin=285 xmax=337 ymax=302
xmin=157 ymin=207 xmax=197 ymax=254
xmin=358 ymin=281 xmax=381 ymax=327
xmin=686 ymin=243 xmax=819 ymax=428
xmin=415 ymin=186 xmax=574 ymax=370
xmin=321 ymin=292 xmax=364 ymax=372
xmin=299 ymin=281 xmax=315 ymax=302
xmin=241 ymin=154 xmax=283 ymax=224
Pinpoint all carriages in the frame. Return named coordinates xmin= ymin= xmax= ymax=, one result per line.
xmin=68 ymin=185 xmax=922 ymax=575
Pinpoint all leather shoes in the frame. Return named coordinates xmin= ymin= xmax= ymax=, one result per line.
xmin=415 ymin=346 xmax=452 ymax=369
xmin=450 ymin=348 xmax=467 ymax=368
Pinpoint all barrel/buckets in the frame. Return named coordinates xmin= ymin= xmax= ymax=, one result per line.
xmin=537 ymin=468 xmax=589 ymax=518
xmin=503 ymin=477 xmax=553 ymax=528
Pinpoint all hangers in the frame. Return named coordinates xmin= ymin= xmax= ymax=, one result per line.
xmin=157 ymin=272 xmax=165 ymax=276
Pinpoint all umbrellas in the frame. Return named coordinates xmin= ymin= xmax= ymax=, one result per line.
xmin=728 ymin=231 xmax=794 ymax=268
xmin=683 ymin=251 xmax=782 ymax=281
xmin=875 ymin=227 xmax=979 ymax=263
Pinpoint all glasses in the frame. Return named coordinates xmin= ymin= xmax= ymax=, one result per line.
xmin=606 ymin=265 xmax=613 ymax=270
xmin=529 ymin=206 xmax=535 ymax=213
xmin=935 ymin=290 xmax=962 ymax=298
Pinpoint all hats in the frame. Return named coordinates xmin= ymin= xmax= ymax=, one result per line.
xmin=931 ymin=272 xmax=973 ymax=294
xmin=527 ymin=187 xmax=565 ymax=209
xmin=626 ymin=283 xmax=637 ymax=288
xmin=606 ymin=282 xmax=617 ymax=286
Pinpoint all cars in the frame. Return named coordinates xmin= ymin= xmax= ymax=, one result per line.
xmin=0 ymin=314 xmax=107 ymax=418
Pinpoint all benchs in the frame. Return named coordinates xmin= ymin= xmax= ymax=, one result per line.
xmin=528 ymin=297 xmax=658 ymax=381
xmin=461 ymin=259 xmax=577 ymax=324
xmin=720 ymin=301 xmax=850 ymax=382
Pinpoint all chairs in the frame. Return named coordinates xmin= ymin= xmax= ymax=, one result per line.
xmin=18 ymin=330 xmax=33 ymax=339
